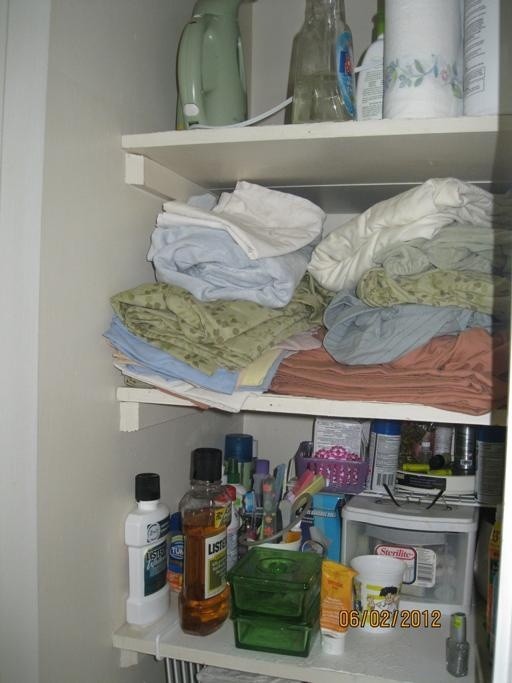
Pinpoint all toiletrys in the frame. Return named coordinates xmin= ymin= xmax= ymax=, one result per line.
xmin=320 ymin=560 xmax=360 ymax=655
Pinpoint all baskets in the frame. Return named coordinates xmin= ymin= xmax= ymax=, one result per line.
xmin=294 ymin=441 xmax=369 ymax=493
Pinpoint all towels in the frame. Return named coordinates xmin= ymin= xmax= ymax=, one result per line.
xmin=102 ymin=173 xmax=512 ymax=417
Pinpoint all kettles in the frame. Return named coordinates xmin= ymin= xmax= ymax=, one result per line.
xmin=170 ymin=0 xmax=249 ymax=130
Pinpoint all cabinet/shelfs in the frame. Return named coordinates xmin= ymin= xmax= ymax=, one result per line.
xmin=109 ymin=111 xmax=511 ymax=682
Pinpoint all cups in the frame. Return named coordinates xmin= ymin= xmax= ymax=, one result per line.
xmin=349 ymin=554 xmax=405 ymax=635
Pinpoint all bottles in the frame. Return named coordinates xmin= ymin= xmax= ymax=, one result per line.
xmin=417 ymin=424 xmax=476 ymax=474
xmin=446 ymin=613 xmax=470 ymax=677
xmin=281 ymin=0 xmax=353 ymax=124
xmin=367 ymin=419 xmax=402 ymax=496
xmin=222 ymin=430 xmax=272 ymax=509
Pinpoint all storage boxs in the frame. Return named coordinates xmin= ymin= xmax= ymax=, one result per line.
xmin=337 ymin=493 xmax=479 ymax=618
